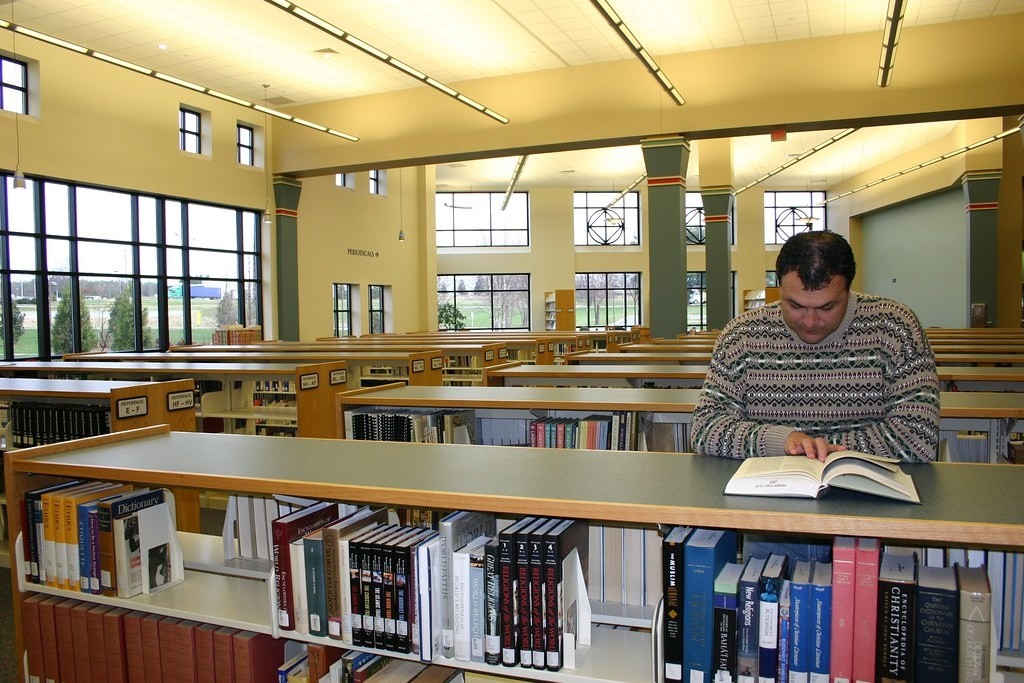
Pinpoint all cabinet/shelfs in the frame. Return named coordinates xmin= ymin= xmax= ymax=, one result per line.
xmin=0 ymin=287 xmax=1024 ymax=683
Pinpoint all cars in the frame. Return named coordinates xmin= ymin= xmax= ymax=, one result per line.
xmin=689 ymin=292 xmax=706 ymax=304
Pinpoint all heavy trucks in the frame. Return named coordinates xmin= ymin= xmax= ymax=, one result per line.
xmin=167 ymin=285 xmax=222 ymax=301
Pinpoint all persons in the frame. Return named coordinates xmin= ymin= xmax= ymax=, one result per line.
xmin=689 ymin=231 xmax=941 ymax=465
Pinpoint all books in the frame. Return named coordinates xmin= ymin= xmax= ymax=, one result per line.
xmin=442 ymin=355 xmax=480 ymax=369
xmin=852 ymin=537 xmax=879 ymax=683
xmin=528 ymin=410 xmax=692 ymax=451
xmin=778 ymin=560 xmax=832 ymax=683
xmin=916 ymin=559 xmax=958 ymax=683
xmin=277 ymin=643 xmax=464 ymax=683
xmin=253 ymin=379 xmax=297 ymax=438
xmin=228 ymin=493 xmax=660 ymax=672
xmin=738 ymin=554 xmax=766 ymax=683
xmin=723 ymin=450 xmax=924 ymax=506
xmin=758 ymin=552 xmax=788 ymax=683
xmin=343 ymin=407 xmax=478 ymax=446
xmin=876 ymin=549 xmax=919 ymax=683
xmin=21 ymin=479 xmax=185 ymax=599
xmin=958 ymin=564 xmax=993 ymax=683
xmin=712 ymin=560 xmax=745 ymax=683
xmin=211 ymin=325 xmax=261 ymax=346
xmin=829 ymin=536 xmax=853 ymax=683
xmin=7 ymin=401 xmax=109 ymax=448
xmin=660 ymin=527 xmax=737 ymax=683
xmin=925 ymin=547 xmax=1024 ymax=658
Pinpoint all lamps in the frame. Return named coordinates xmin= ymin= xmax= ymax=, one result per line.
xmin=12 ymin=0 xmax=25 ymax=188
xmin=0 ymin=0 xmax=509 ymax=142
xmin=263 ymin=84 xmax=271 ymax=224
xmin=501 ymin=154 xmax=530 ymax=210
xmin=590 ymin=0 xmax=686 ymax=107
xmin=605 ymin=171 xmax=647 ymax=209
xmin=877 ymin=0 xmax=908 ymax=87
xmin=731 ymin=128 xmax=1020 ymax=205
xmin=399 ymin=169 xmax=405 ymax=241
